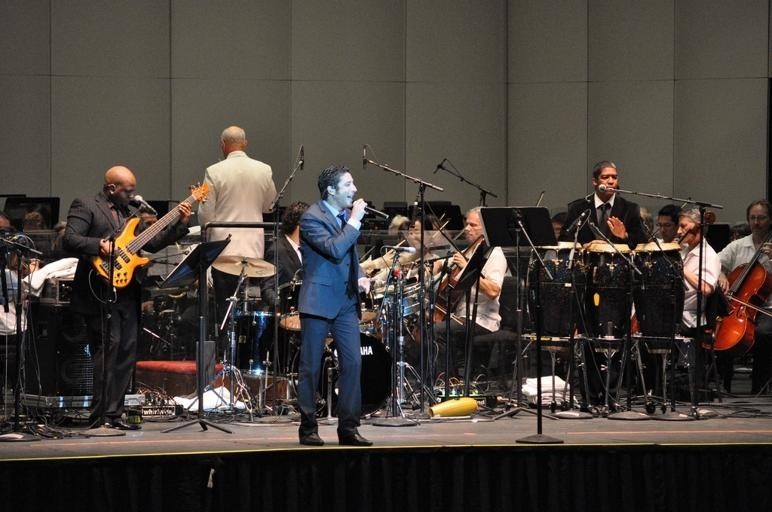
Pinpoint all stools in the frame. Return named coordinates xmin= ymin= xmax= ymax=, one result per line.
xmin=533 ymin=339 xmax=675 ymax=416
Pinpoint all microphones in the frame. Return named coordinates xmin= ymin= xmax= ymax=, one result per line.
xmin=360 ymin=144 xmax=370 ymax=170
xmin=132 ymin=193 xmax=157 ymax=216
xmin=299 ymin=144 xmax=306 ymax=173
xmin=353 ymin=197 xmax=391 ymax=221
xmin=433 ymin=156 xmax=447 ymax=175
xmin=598 ymin=183 xmax=608 ymax=194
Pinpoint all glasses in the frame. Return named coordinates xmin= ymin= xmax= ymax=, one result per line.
xmin=656 ymin=223 xmax=677 ymax=227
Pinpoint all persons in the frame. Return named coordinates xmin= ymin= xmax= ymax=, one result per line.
xmin=1 ymin=211 xmax=50 ymax=391
xmin=63 ymin=164 xmax=193 ymax=429
xmin=393 ymin=219 xmax=447 ymax=281
xmin=715 ymin=197 xmax=772 ymax=388
xmin=196 ymin=125 xmax=277 ymax=368
xmin=259 ymin=201 xmax=312 ymax=371
xmin=297 ymin=162 xmax=372 ymax=447
xmin=543 ymin=159 xmax=721 ymax=412
xmin=423 ymin=206 xmax=512 ymax=381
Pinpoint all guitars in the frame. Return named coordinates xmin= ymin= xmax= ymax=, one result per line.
xmin=92 ymin=182 xmax=210 ymax=289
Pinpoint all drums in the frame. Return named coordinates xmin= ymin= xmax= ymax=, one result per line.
xmin=292 ymin=336 xmax=393 ymax=420
xmin=634 ymin=243 xmax=683 ymax=355
xmin=583 ymin=243 xmax=632 ymax=352
xmin=526 ymin=242 xmax=581 ymax=351
xmin=385 ymin=283 xmax=425 ymax=319
xmin=276 ymin=278 xmax=378 ymax=331
xmin=232 ymin=312 xmax=282 ymax=374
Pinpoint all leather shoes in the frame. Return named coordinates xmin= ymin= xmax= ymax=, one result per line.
xmin=337 ymin=433 xmax=373 ymax=446
xmin=299 ymin=432 xmax=324 ymax=446
xmin=104 ymin=417 xmax=142 ymax=430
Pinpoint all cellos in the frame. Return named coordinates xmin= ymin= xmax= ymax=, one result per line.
xmin=407 ymin=241 xmax=490 ymax=345
xmin=697 ymin=229 xmax=771 ymax=356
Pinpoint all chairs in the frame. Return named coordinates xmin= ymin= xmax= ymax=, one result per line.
xmin=456 ymin=277 xmax=524 ymax=406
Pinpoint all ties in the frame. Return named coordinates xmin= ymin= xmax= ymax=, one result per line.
xmin=337 ymin=214 xmax=355 ymax=300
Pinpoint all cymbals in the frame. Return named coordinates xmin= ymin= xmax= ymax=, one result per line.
xmin=212 ymin=256 xmax=278 ymax=278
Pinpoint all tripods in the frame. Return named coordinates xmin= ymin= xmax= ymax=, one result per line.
xmin=480 ymin=220 xmax=560 ymax=421
xmin=160 ymin=260 xmax=235 ymax=434
xmin=369 ymin=157 xmax=444 ymax=408
